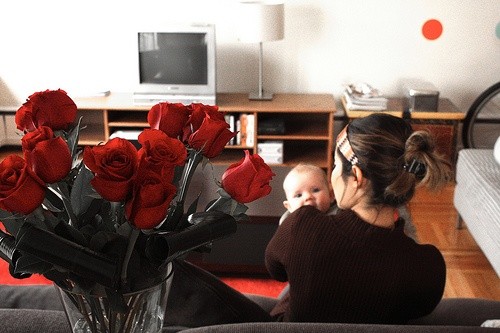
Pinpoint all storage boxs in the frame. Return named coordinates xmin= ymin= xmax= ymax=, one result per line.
xmin=408 ymin=90 xmax=439 ymax=112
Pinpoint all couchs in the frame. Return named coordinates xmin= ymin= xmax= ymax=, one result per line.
xmin=0 ymin=285 xmax=500 ymax=333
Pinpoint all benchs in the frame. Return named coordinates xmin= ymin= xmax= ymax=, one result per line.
xmin=451 ymin=134 xmax=500 ymax=278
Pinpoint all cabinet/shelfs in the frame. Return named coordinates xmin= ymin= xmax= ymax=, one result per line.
xmin=0 ymin=92 xmax=337 ymax=184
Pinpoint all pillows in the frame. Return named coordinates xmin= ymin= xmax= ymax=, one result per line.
xmin=164 ymin=262 xmax=274 ymax=328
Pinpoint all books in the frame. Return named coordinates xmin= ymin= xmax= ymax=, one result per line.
xmin=224 ymin=114 xmax=254 ymax=145
xmin=343 ymin=82 xmax=388 ymax=110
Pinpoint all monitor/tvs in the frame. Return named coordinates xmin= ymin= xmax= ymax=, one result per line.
xmin=133 ymin=24 xmax=216 ymax=107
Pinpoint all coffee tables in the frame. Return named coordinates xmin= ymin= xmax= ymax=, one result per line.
xmin=179 ymin=164 xmax=329 ymax=277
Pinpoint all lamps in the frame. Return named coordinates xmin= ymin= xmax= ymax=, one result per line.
xmin=237 ymin=1 xmax=284 ymax=101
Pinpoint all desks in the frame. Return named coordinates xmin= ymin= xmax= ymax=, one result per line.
xmin=334 ymin=96 xmax=466 ymax=164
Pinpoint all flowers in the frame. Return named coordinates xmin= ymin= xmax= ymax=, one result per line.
xmin=0 ymin=88 xmax=277 ymax=333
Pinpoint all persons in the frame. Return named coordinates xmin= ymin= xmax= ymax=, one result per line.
xmin=276 ymin=164 xmax=338 ymax=225
xmin=265 ymin=114 xmax=446 ymax=325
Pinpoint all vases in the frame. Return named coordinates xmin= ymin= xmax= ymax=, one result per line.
xmin=53 ymin=261 xmax=174 ymax=333
